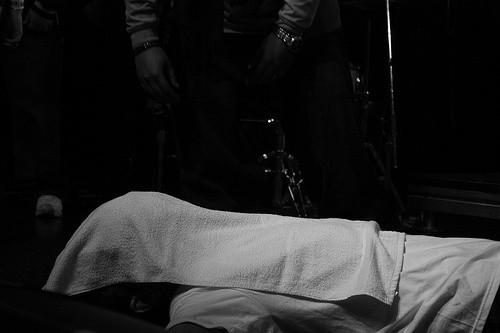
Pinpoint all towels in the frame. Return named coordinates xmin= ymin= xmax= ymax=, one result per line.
xmin=42 ymin=191 xmax=407 ymax=306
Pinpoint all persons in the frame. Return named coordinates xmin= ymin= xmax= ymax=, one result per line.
xmin=0 ymin=0 xmax=500 ymax=333
